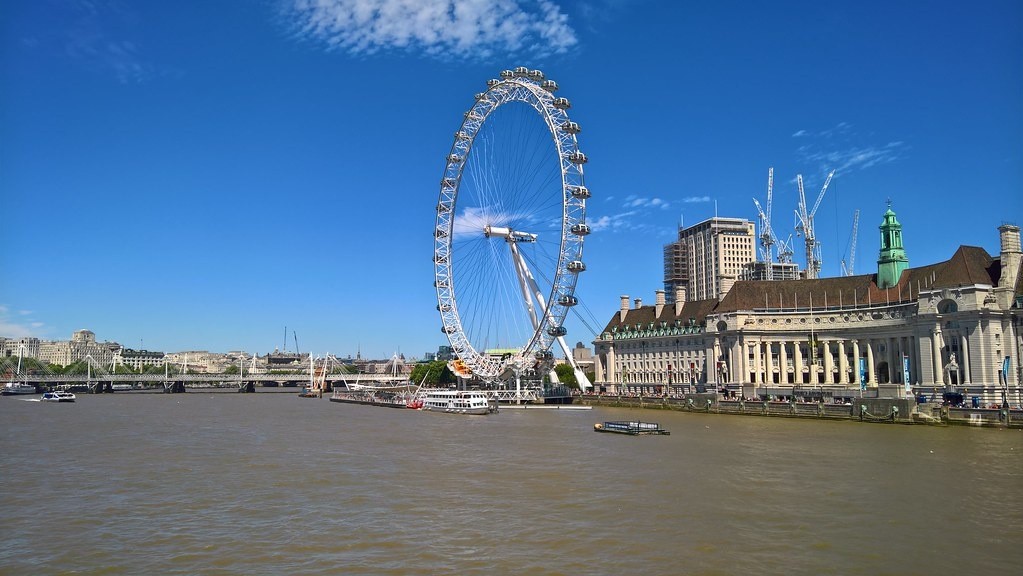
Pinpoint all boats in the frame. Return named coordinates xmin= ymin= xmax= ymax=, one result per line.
xmin=420 ymin=388 xmax=489 ymax=414
xmin=497 ymin=355 xmax=521 ymax=381
xmin=329 ymin=390 xmax=375 ymax=405
xmin=593 ymin=419 xmax=670 ymax=436
xmin=446 ymin=359 xmax=472 ymax=379
xmin=297 ymin=388 xmax=319 ymax=398
xmin=217 ymin=382 xmax=240 ymax=389
xmin=348 ymin=384 xmax=422 ymax=409
xmin=191 ymin=381 xmax=214 ymax=389
xmin=39 ymin=391 xmax=76 ymax=403
xmin=0 ymin=383 xmax=36 ymax=395
xmin=111 ymin=384 xmax=131 ymax=391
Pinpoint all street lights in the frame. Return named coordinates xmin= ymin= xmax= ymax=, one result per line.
xmin=1001 ymin=384 xmax=1009 ymax=408
xmin=942 ymin=385 xmax=950 ymax=406
xmin=741 ymin=384 xmax=746 ymax=400
xmin=574 ymin=380 xmax=576 ymax=391
xmin=765 ymin=385 xmax=769 ymax=400
xmin=820 ymin=384 xmax=824 ymax=402
xmin=792 ymin=384 xmax=797 ymax=402
